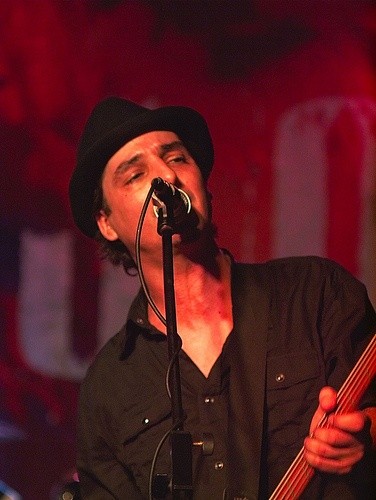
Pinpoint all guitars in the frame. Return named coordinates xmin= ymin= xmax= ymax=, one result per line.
xmin=266 ymin=331 xmax=375 ymax=500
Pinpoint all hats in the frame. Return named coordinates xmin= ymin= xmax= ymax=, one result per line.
xmin=71 ymin=97 xmax=217 ymax=246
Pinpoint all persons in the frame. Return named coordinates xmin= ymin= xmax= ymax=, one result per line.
xmin=55 ymin=96 xmax=376 ymax=500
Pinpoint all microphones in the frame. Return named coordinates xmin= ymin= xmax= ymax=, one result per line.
xmin=151 ymin=178 xmax=192 ymax=221
